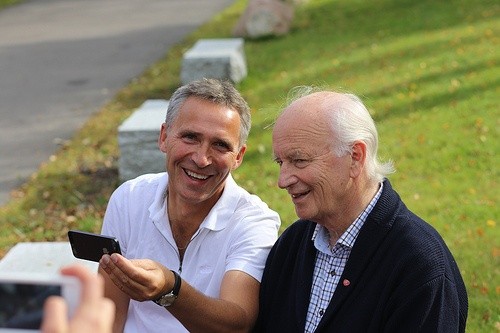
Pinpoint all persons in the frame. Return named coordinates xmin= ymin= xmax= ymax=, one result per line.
xmin=254 ymin=91 xmax=469 ymax=333
xmin=39 ymin=264 xmax=129 ymax=333
xmin=95 ymin=79 xmax=281 ymax=333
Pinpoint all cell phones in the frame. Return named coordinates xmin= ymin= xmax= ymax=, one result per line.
xmin=0 ymin=273 xmax=81 ymax=333
xmin=67 ymin=230 xmax=123 ymax=263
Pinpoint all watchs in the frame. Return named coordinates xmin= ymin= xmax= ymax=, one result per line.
xmin=152 ymin=270 xmax=181 ymax=307
